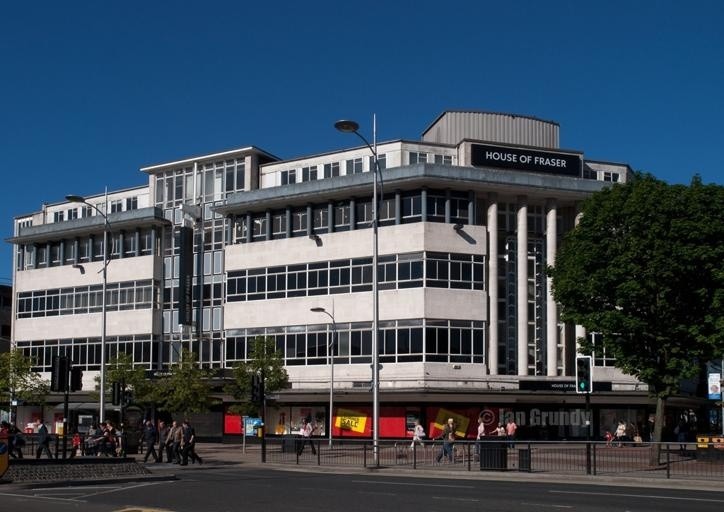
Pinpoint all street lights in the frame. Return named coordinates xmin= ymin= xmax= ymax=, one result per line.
xmin=309 ymin=298 xmax=335 ymax=450
xmin=65 ymin=186 xmax=116 ymax=430
xmin=335 ymin=113 xmax=385 ymax=470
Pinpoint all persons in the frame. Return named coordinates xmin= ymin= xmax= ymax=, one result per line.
xmin=473 ymin=418 xmax=486 ymax=462
xmin=410 ymin=419 xmax=427 ymax=450
xmin=0 ymin=419 xmax=205 ymax=467
xmin=505 ymin=419 xmax=518 ymax=449
xmin=674 ymin=419 xmax=689 ymax=457
xmin=489 ymin=421 xmax=506 ymax=437
xmin=433 ymin=418 xmax=457 ymax=464
xmin=603 ymin=419 xmax=643 ymax=448
xmin=296 ymin=417 xmax=317 ymax=456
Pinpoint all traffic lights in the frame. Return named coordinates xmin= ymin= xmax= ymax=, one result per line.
xmin=574 ymin=355 xmax=593 ymax=395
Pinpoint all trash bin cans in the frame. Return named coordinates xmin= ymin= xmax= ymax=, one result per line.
xmin=480 ymin=435 xmax=507 ymax=471
